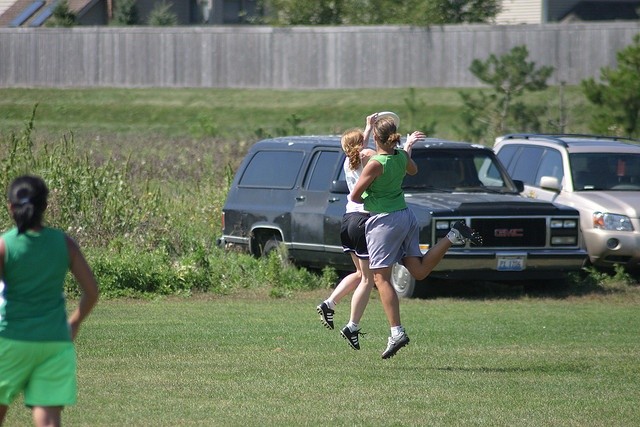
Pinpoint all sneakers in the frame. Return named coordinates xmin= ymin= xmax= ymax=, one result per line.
xmin=381 ymin=328 xmax=409 ymax=357
xmin=340 ymin=324 xmax=366 ymax=351
xmin=450 ymin=222 xmax=485 ymax=246
xmin=317 ymin=301 xmax=335 ymax=330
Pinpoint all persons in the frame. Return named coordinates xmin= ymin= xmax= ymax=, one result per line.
xmin=351 ymin=118 xmax=485 ymax=360
xmin=316 ymin=113 xmax=427 ymax=350
xmin=0 ymin=176 xmax=99 ymax=427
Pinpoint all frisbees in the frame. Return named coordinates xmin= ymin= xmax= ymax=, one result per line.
xmin=373 ymin=111 xmax=400 ymax=129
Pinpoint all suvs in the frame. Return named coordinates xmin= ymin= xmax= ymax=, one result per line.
xmin=479 ymin=135 xmax=640 ymax=267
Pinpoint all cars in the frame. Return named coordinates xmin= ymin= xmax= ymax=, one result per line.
xmin=220 ymin=135 xmax=588 ymax=299
